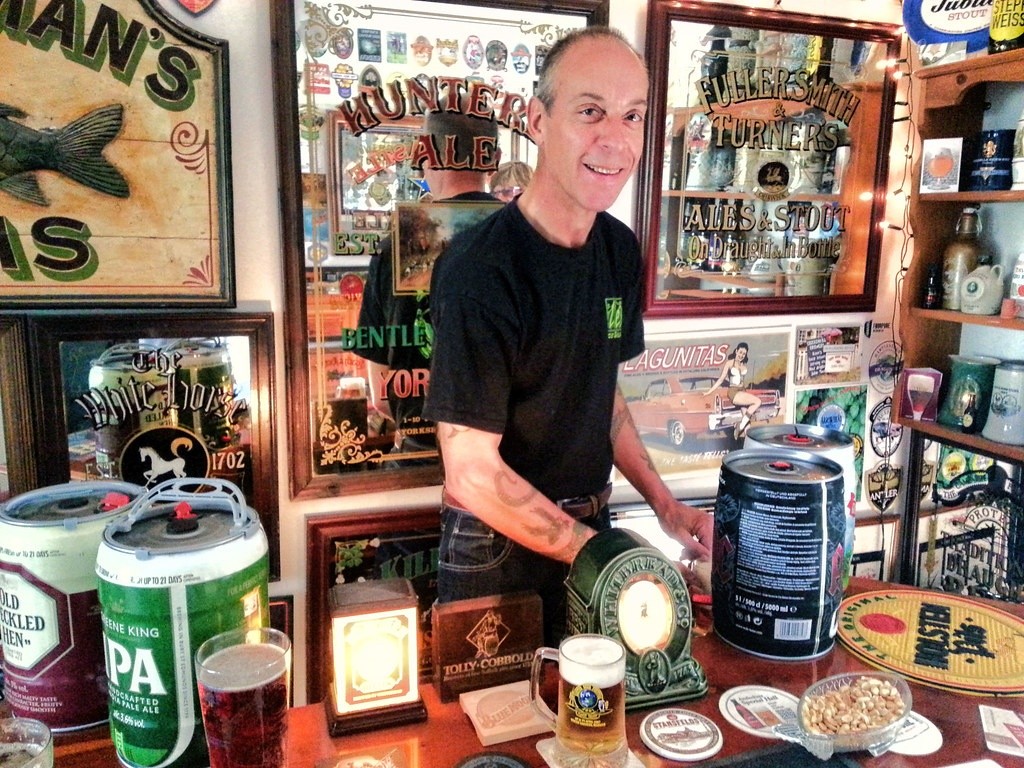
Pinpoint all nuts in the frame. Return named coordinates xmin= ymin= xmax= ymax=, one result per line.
xmin=802 ymin=675 xmax=905 ymax=737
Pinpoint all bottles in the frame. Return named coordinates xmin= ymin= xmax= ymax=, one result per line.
xmin=941 ymin=203 xmax=987 ymax=312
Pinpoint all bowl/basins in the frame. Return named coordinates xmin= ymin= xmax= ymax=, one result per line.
xmin=797 ymin=672 xmax=912 ymax=751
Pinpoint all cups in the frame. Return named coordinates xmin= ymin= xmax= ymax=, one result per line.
xmin=531 ymin=632 xmax=630 ymax=767
xmin=194 ymin=623 xmax=291 ymax=768
xmin=0 ymin=717 xmax=56 ymax=768
xmin=961 ymin=130 xmax=1015 ymax=190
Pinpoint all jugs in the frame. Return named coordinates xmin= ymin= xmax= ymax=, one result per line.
xmin=962 ymin=254 xmax=1004 ymax=315
xmin=932 ymin=354 xmax=1000 ymax=430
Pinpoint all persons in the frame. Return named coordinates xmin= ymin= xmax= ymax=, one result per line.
xmin=418 ymin=27 xmax=714 ymax=648
xmin=351 ymin=95 xmax=508 ymax=469
xmin=488 ymin=161 xmax=534 ymax=203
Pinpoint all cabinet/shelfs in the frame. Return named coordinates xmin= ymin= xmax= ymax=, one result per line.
xmin=658 ymin=76 xmax=881 ymax=300
xmin=884 ymin=48 xmax=1024 ymax=464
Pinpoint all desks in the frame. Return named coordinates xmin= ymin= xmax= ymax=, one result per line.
xmin=0 ymin=570 xmax=1024 ymax=768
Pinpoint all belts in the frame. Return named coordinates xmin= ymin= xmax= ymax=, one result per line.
xmin=441 ymin=484 xmax=614 ymax=523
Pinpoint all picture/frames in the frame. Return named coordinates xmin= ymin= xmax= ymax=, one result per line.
xmin=0 ymin=309 xmax=283 ymax=583
xmin=303 ymin=507 xmax=442 ymax=703
xmin=267 ymin=0 xmax=611 ymax=504
xmin=0 ymin=0 xmax=243 ymax=306
xmin=638 ymin=0 xmax=902 ymax=314
xmin=325 ymin=101 xmax=431 ymax=251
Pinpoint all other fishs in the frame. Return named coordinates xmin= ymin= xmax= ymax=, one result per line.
xmin=0 ymin=101 xmax=131 ymax=207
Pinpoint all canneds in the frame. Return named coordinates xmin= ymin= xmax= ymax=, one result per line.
xmin=710 ymin=422 xmax=858 ymax=659
xmin=0 ymin=482 xmax=269 ymax=768
xmin=86 ymin=336 xmax=234 ymax=478
